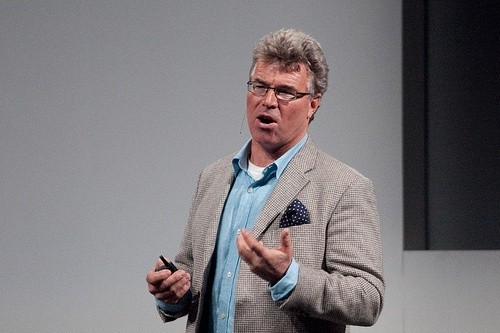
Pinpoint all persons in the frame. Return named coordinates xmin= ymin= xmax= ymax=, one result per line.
xmin=146 ymin=29 xmax=385 ymax=333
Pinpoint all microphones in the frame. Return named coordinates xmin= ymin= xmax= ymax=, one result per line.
xmin=240 ymin=111 xmax=246 ymax=134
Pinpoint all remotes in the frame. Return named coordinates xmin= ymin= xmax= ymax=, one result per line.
xmin=155 ymin=255 xmax=178 ymax=278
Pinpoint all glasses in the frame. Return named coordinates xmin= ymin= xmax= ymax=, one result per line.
xmin=247 ymin=80 xmax=311 ymax=101
